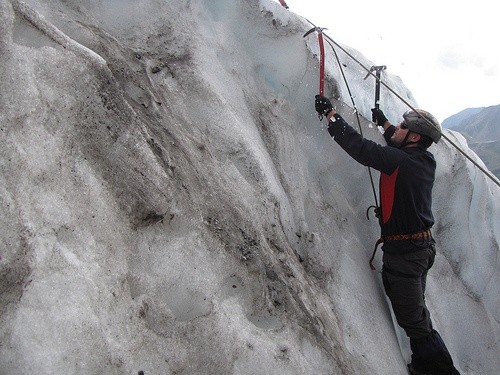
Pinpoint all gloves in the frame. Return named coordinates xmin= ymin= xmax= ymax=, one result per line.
xmin=371 ymin=107 xmax=388 ymax=126
xmin=314 ymin=94 xmax=334 ymax=117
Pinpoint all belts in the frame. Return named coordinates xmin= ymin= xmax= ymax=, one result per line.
xmin=381 ymin=229 xmax=432 ymax=242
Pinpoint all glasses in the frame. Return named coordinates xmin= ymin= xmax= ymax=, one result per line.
xmin=400 ymin=122 xmax=409 ymax=129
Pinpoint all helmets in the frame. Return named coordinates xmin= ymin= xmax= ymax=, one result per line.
xmin=402 ymin=108 xmax=442 ymax=144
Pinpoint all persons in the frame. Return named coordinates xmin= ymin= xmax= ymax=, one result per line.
xmin=314 ymin=94 xmax=460 ymax=375
